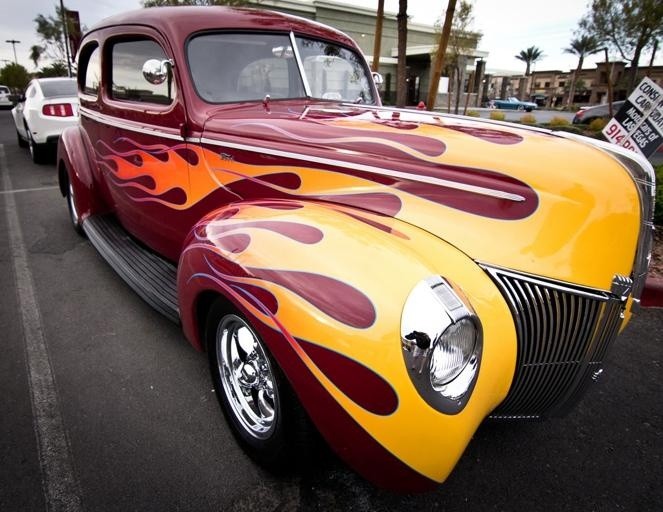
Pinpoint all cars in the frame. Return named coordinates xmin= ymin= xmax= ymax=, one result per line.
xmin=490 ymin=95 xmax=538 ymax=112
xmin=11 ymin=78 xmax=81 ymax=165
xmin=571 ymin=100 xmax=662 ymax=168
xmin=55 ymin=0 xmax=659 ymax=497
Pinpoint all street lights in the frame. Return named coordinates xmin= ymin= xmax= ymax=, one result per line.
xmin=0 ymin=59 xmax=10 ymax=66
xmin=5 ymin=39 xmax=21 ymax=66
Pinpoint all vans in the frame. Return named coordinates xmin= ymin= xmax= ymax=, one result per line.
xmin=0 ymin=85 xmax=18 ymax=109
